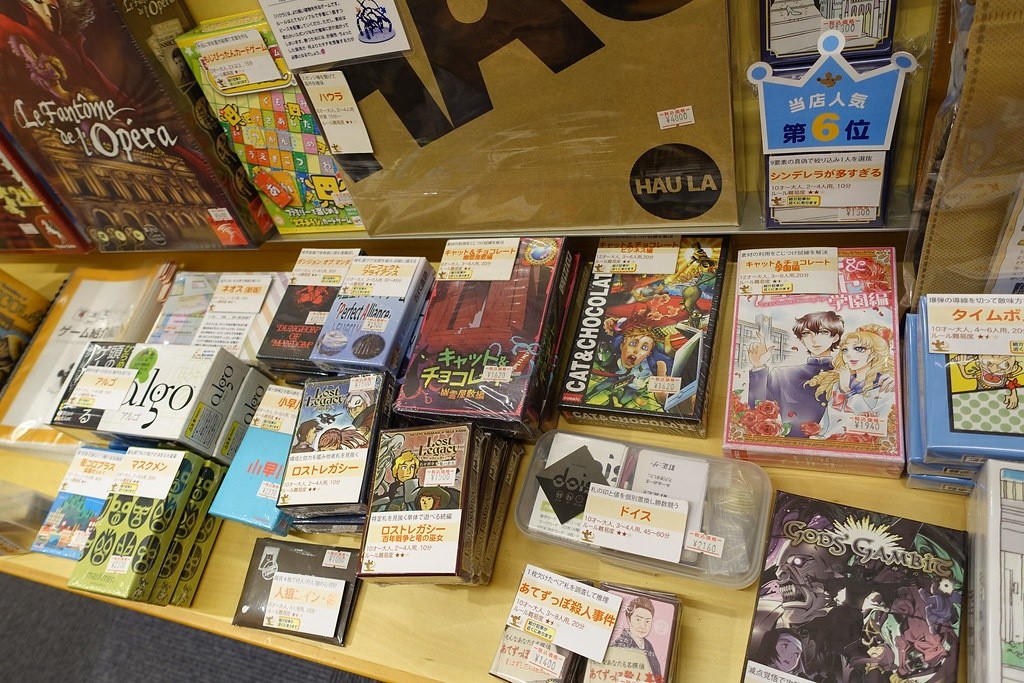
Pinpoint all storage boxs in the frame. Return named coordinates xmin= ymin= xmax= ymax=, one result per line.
xmin=31 ymin=232 xmax=1024 ymax=681
xmin=0 ymin=0 xmax=367 ymax=246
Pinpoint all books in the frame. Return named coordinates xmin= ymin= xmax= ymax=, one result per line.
xmin=0 ymin=265 xmax=164 ymax=446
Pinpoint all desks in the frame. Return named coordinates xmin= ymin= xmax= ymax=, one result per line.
xmin=0 ymin=247 xmax=1024 ymax=683
